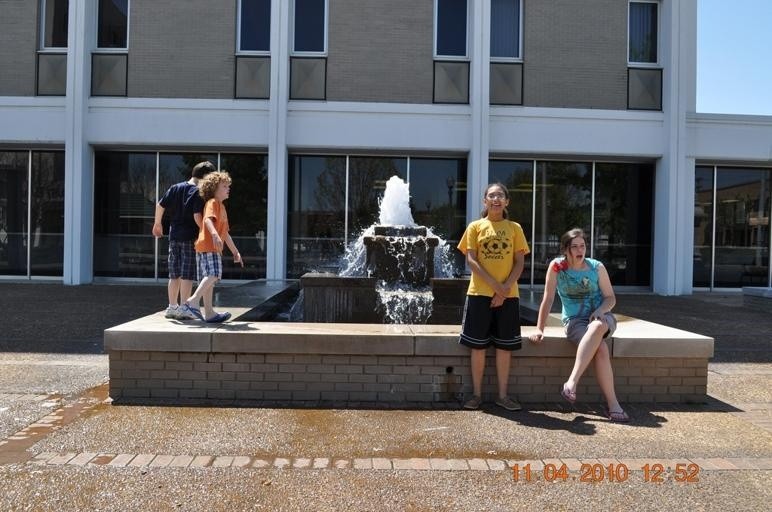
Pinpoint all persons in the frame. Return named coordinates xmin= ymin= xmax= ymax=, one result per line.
xmin=457 ymin=183 xmax=530 ymax=411
xmin=185 ymin=172 xmax=244 ymax=323
xmin=152 ymin=161 xmax=216 ymax=320
xmin=528 ymin=229 xmax=631 ymax=422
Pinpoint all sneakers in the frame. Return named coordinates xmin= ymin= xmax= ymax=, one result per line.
xmin=164 ymin=304 xmax=195 ymax=320
xmin=494 ymin=395 xmax=523 ymax=412
xmin=184 ymin=301 xmax=206 ymax=323
xmin=206 ymin=312 xmax=232 ymax=324
xmin=463 ymin=394 xmax=484 ymax=411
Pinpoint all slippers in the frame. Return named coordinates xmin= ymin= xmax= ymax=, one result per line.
xmin=560 ymin=381 xmax=578 ymax=405
xmin=604 ymin=407 xmax=631 ymax=423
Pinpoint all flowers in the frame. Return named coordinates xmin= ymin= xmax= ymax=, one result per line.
xmin=552 ymin=259 xmax=569 ymax=273
